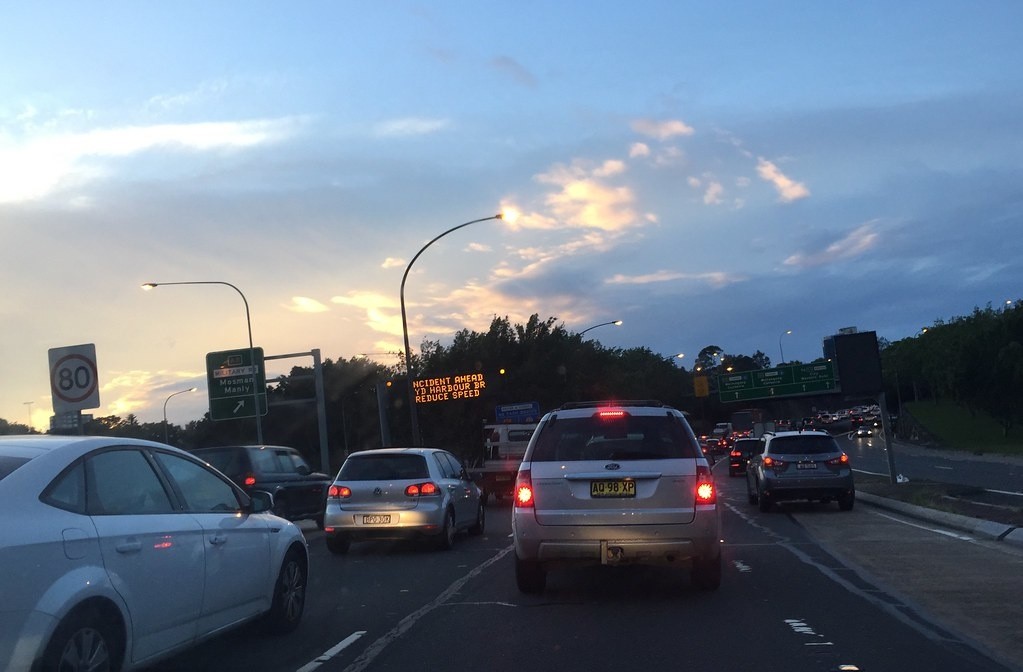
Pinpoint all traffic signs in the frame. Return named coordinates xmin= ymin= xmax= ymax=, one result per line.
xmin=717 ymin=360 xmax=837 ymax=402
xmin=204 ymin=347 xmax=269 ymax=422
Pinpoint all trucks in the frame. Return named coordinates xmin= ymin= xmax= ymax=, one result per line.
xmin=468 ymin=421 xmax=538 ymax=502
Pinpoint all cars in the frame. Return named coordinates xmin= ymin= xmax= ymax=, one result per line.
xmin=323 ymin=447 xmax=486 ymax=553
xmin=0 ymin=435 xmax=313 ymax=672
xmin=578 ymin=403 xmax=882 ymax=479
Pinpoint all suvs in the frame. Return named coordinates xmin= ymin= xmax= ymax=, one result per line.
xmin=741 ymin=427 xmax=856 ymax=514
xmin=512 ymin=402 xmax=726 ymax=595
xmin=185 ymin=443 xmax=334 ymax=530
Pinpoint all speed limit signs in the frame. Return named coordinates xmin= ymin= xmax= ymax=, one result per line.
xmin=50 ymin=353 xmax=99 ymax=403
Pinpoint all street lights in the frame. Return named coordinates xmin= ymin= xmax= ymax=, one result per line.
xmin=565 ymin=319 xmax=623 ymax=402
xmin=399 ymin=207 xmax=522 ymax=450
xmin=163 ymin=387 xmax=198 ymax=444
xmin=139 ymin=281 xmax=265 ymax=444
xmin=779 ymin=330 xmax=792 ymax=363
